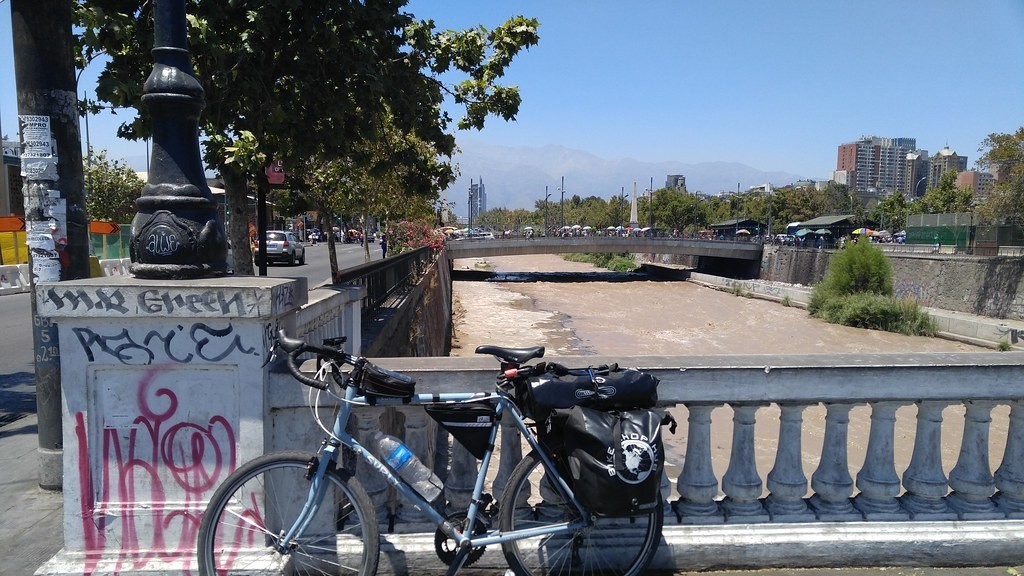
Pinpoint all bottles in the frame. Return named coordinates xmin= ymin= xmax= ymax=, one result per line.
xmin=374 ymin=430 xmax=443 ymax=503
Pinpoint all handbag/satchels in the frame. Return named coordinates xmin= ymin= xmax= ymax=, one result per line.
xmin=556 ymin=409 xmax=682 ymax=517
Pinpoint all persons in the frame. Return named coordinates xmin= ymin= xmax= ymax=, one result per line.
xmin=700 ymin=233 xmax=905 ymax=250
xmin=933 ymin=234 xmax=940 ymax=253
xmin=379 ymin=235 xmax=387 ymax=258
xmin=675 ymin=228 xmax=679 ymax=237
xmin=527 ymin=228 xmax=644 ymax=240
xmin=308 ymin=231 xmax=364 ymax=247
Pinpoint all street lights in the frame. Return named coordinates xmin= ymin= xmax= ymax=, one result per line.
xmin=620 ymin=187 xmax=629 ymax=228
xmin=916 ymin=176 xmax=927 ymax=198
xmin=543 ymin=185 xmax=554 ymax=235
xmin=557 ymin=175 xmax=565 ymax=232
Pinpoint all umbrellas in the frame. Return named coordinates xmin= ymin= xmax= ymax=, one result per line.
xmin=561 ymin=225 xmax=650 ymax=232
xmin=852 ymin=228 xmax=906 ymax=237
xmin=815 ymin=228 xmax=832 ymax=235
xmin=736 ymin=229 xmax=750 ymax=237
xmin=798 ymin=228 xmax=814 ymax=236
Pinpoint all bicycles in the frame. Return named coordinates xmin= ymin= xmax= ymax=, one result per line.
xmin=198 ymin=326 xmax=680 ymax=576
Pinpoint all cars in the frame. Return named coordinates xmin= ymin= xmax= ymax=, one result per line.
xmin=475 ymin=232 xmax=494 ymax=241
xmin=253 ymin=230 xmax=306 ymax=265
xmin=455 ymin=233 xmax=485 ymax=242
